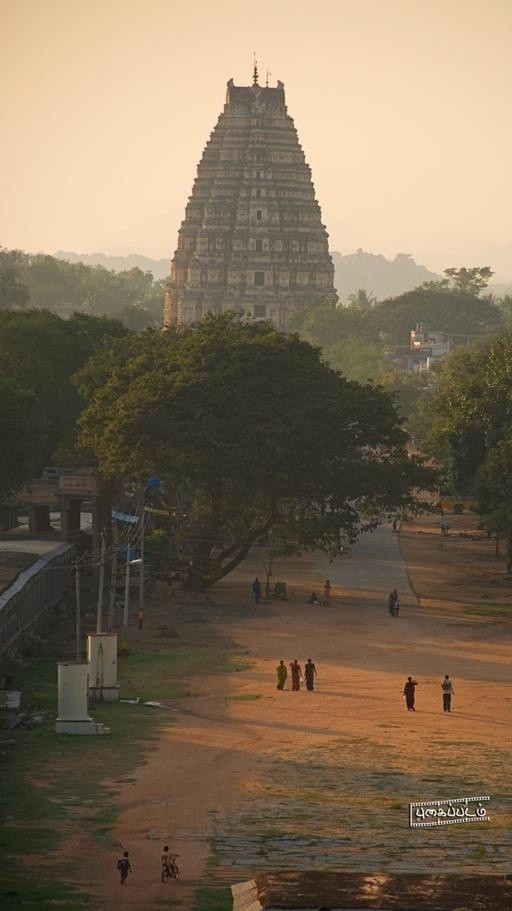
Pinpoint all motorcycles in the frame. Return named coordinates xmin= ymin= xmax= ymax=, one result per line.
xmin=391 ymin=600 xmax=399 ymax=617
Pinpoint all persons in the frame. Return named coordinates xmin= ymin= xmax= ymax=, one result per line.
xmin=290 ymin=663 xmax=294 ymax=666
xmin=309 ymin=591 xmax=323 ymax=607
xmin=387 ymin=588 xmax=398 ymax=614
xmin=116 ymin=851 xmax=133 ymax=885
xmin=275 ymin=661 xmax=288 ymax=690
xmin=323 ymin=579 xmax=331 ymax=601
xmin=304 ymin=658 xmax=317 ymax=691
xmin=438 ymin=522 xmax=450 ymax=536
xmin=391 ymin=521 xmax=396 ymax=530
xmin=160 ymin=845 xmax=180 ymax=879
xmin=402 ymin=676 xmax=418 ymax=711
xmin=291 ymin=660 xmax=302 ymax=691
xmin=252 ymin=578 xmax=261 ymax=603
xmin=440 ymin=674 xmax=455 ymax=712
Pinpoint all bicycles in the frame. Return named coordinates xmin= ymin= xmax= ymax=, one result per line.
xmin=441 ymin=528 xmax=448 ymax=536
xmin=162 ymin=859 xmax=179 ymax=882
